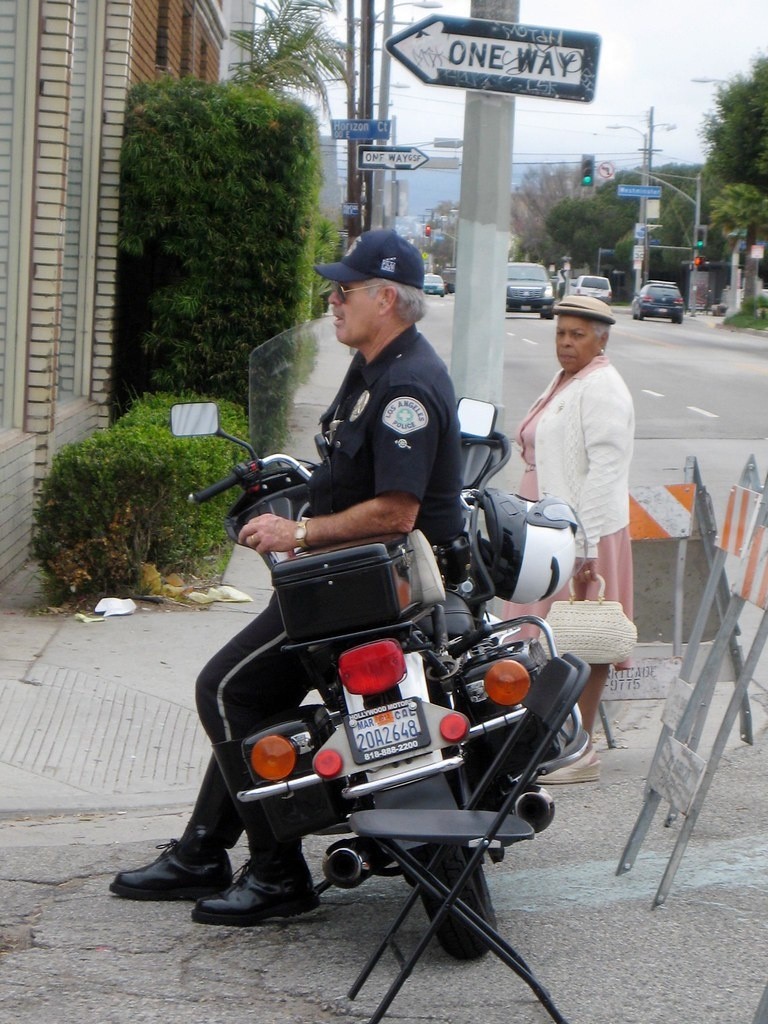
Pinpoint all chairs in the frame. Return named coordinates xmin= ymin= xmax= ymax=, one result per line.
xmin=347 ymin=653 xmax=590 ymax=1024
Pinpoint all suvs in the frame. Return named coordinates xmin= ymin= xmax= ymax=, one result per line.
xmin=441 ymin=267 xmax=456 ymax=294
xmin=570 ymin=275 xmax=612 ymax=305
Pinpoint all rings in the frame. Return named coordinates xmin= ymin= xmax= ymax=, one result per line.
xmin=583 ymin=570 xmax=591 ymax=576
xmin=251 ymin=535 xmax=256 ymax=546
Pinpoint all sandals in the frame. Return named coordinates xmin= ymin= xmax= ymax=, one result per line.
xmin=536 ymin=749 xmax=601 ymax=784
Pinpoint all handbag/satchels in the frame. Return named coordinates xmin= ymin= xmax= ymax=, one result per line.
xmin=538 ymin=573 xmax=638 ymax=664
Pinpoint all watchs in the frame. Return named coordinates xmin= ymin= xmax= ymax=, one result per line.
xmin=294 ymin=518 xmax=312 ymax=550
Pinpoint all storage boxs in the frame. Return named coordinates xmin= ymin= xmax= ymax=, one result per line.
xmin=459 ymin=637 xmax=570 ymax=778
xmin=241 ymin=704 xmax=355 ymax=842
xmin=271 ymin=533 xmax=423 ymax=641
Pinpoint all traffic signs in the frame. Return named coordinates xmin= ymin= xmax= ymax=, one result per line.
xmin=356 ymin=145 xmax=431 ymax=171
xmin=384 ymin=13 xmax=602 ymax=105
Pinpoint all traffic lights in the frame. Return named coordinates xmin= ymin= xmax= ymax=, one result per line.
xmin=582 ymin=154 xmax=594 ymax=186
xmin=426 ymin=226 xmax=431 ymax=236
xmin=695 ymin=256 xmax=705 ymax=266
xmin=697 ymin=228 xmax=704 ymax=248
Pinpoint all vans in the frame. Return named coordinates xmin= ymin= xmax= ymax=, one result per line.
xmin=506 ymin=262 xmax=554 ymax=320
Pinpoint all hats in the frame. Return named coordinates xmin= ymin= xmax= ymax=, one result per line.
xmin=313 ymin=227 xmax=425 ymax=289
xmin=553 ymin=295 xmax=617 ymax=325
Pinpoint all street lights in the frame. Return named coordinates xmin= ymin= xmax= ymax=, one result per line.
xmin=606 ymin=125 xmax=648 ymax=296
xmin=344 ymin=0 xmax=443 ymax=231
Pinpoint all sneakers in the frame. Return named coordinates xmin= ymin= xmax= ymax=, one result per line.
xmin=191 ymin=857 xmax=321 ymax=925
xmin=109 ymin=838 xmax=233 ymax=900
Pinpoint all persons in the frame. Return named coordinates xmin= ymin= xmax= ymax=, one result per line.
xmin=503 ymin=295 xmax=634 ymax=785
xmin=108 ymin=228 xmax=471 ymax=927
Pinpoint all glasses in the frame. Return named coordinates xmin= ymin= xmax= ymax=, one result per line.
xmin=332 ymin=279 xmax=383 ymax=304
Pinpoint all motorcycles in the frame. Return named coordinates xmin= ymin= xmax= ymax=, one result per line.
xmin=169 ymin=397 xmax=593 ymax=963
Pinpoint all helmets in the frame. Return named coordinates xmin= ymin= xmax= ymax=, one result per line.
xmin=476 ymin=488 xmax=577 ymax=604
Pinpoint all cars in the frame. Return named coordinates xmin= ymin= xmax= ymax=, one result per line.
xmin=631 ymin=280 xmax=684 ymax=324
xmin=423 ymin=273 xmax=445 ymax=297
